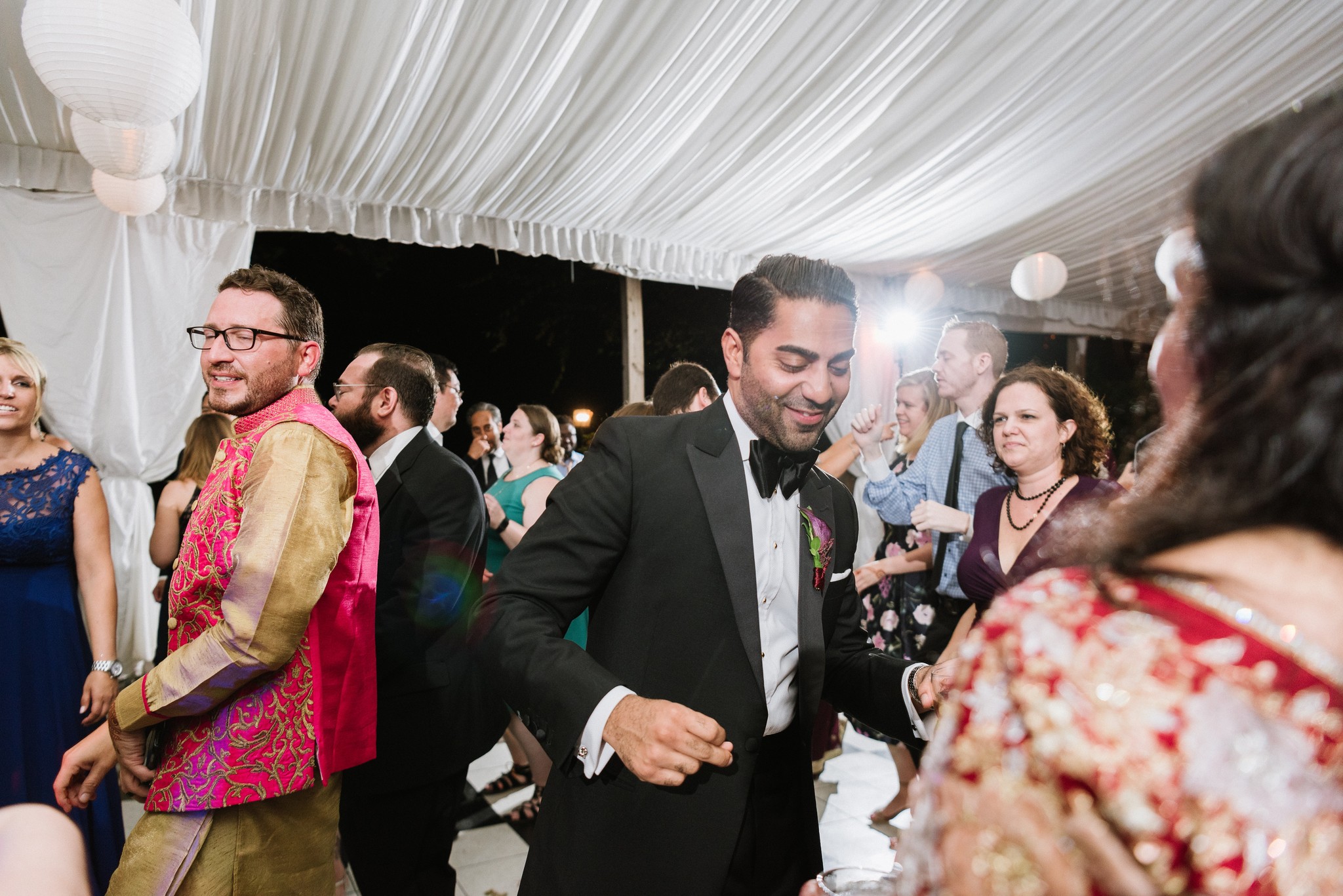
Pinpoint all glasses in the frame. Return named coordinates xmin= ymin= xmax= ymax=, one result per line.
xmin=439 ymin=382 xmax=464 ymax=398
xmin=186 ymin=324 xmax=307 ymax=352
xmin=331 ymin=382 xmax=386 ymax=402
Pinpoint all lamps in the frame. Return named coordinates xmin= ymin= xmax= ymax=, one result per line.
xmin=1153 ymin=225 xmax=1208 ymax=286
xmin=1010 ymin=252 xmax=1068 ymax=301
xmin=901 ymin=272 xmax=945 ymax=312
xmin=69 ymin=108 xmax=176 ymax=181
xmin=22 ymin=0 xmax=203 ymax=129
xmin=92 ymin=167 xmax=168 ymax=216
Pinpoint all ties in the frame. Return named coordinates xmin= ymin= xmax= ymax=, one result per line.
xmin=487 ymin=453 xmax=498 ymax=489
xmin=930 ymin=422 xmax=970 ymax=590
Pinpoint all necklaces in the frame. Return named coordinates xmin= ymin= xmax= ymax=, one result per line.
xmin=1006 ymin=473 xmax=1078 ymax=530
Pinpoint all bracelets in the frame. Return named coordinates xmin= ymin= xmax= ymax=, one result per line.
xmin=495 ymin=516 xmax=509 ymax=535
xmin=908 ymin=664 xmax=934 ymax=710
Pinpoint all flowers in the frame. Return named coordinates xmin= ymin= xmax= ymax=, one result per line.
xmin=796 ymin=501 xmax=832 ymax=592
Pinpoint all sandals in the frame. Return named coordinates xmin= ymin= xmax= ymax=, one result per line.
xmin=872 ymin=780 xmax=912 ymax=823
xmin=482 ymin=762 xmax=535 ymax=793
xmin=503 ymin=785 xmax=545 ymax=824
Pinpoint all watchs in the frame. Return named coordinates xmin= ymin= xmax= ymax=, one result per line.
xmin=91 ymin=660 xmax=123 ymax=679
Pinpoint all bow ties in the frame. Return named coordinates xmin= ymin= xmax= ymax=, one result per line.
xmin=748 ymin=436 xmax=820 ymax=500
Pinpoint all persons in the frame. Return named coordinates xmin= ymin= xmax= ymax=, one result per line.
xmin=802 ymin=93 xmax=1343 ymax=896
xmin=466 ymin=252 xmax=943 ymax=896
xmin=0 ymin=334 xmax=126 ymax=890
xmin=918 ymin=367 xmax=1130 ymax=718
xmin=56 ymin=266 xmax=382 ymax=895
xmin=33 ymin=345 xmax=945 ymax=820
xmin=321 ymin=347 xmax=489 ymax=896
